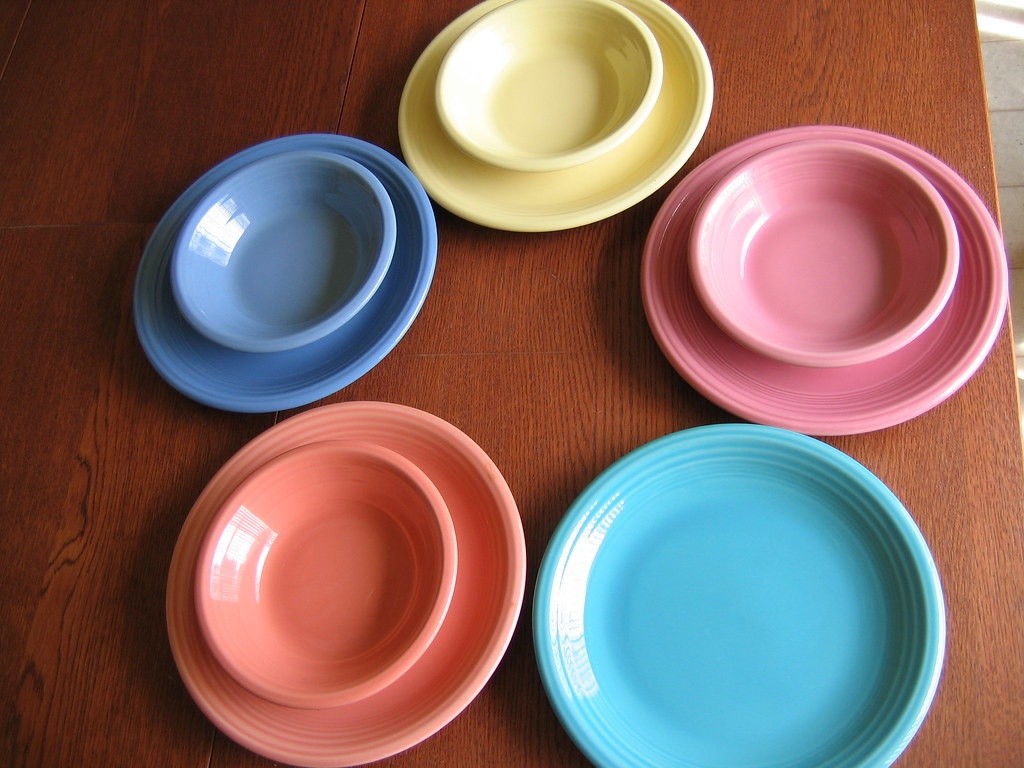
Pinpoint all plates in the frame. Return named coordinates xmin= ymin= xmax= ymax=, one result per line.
xmin=689 ymin=139 xmax=962 ymax=366
xmin=132 ymin=134 xmax=439 ymax=411
xmin=433 ymin=0 xmax=662 ymax=174
xmin=398 ymin=0 xmax=714 ymax=231
xmin=194 ymin=441 xmax=458 ymax=710
xmin=643 ymin=127 xmax=1010 ymax=436
xmin=166 ymin=402 xmax=528 ymax=768
xmin=533 ymin=423 xmax=946 ymax=768
xmin=171 ymin=152 xmax=396 ymax=351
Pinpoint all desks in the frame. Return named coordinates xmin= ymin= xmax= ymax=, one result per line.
xmin=0 ymin=0 xmax=1024 ymax=768
